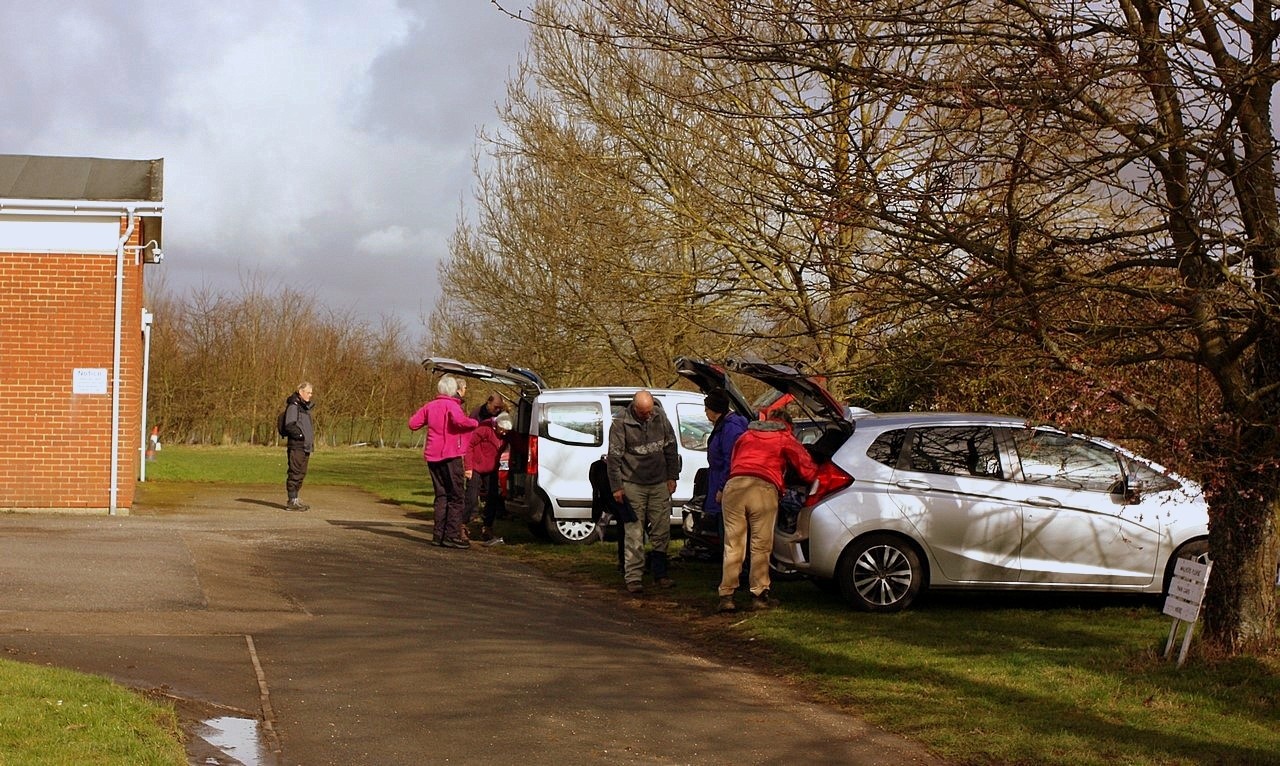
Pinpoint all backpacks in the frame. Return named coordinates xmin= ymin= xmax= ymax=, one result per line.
xmin=278 ymin=402 xmax=300 ymax=437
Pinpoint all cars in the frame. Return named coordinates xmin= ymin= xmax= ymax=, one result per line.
xmin=478 ymin=450 xmax=509 ymax=520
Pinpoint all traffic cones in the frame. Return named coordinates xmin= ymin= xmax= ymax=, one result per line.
xmin=145 ymin=426 xmax=159 ymax=462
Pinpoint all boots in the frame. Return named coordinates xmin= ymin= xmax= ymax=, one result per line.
xmin=720 ymin=596 xmax=737 ymax=613
xmin=752 ymin=590 xmax=780 ymax=609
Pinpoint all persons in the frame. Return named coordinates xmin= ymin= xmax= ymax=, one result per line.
xmin=608 ymin=390 xmax=679 ymax=590
xmin=409 ymin=373 xmax=480 ymax=549
xmin=453 ymin=376 xmax=513 ymax=546
xmin=704 ymin=392 xmax=750 ymax=591
xmin=283 ymin=381 xmax=315 ymax=512
xmin=716 ymin=410 xmax=819 ymax=611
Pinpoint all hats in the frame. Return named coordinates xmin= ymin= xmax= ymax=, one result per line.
xmin=704 ymin=389 xmax=729 ymax=413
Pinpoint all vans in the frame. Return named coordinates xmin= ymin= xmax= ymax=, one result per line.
xmin=420 ymin=357 xmax=738 ymax=546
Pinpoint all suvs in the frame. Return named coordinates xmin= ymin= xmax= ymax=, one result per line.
xmin=668 ymin=355 xmax=874 ymax=542
xmin=722 ymin=357 xmax=1211 ymax=616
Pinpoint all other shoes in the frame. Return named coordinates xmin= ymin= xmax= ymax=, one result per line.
xmin=461 ymin=524 xmax=469 ymax=540
xmin=286 ymin=498 xmax=306 ymax=511
xmin=295 ymin=497 xmax=310 ymax=509
xmin=481 ymin=526 xmax=494 ymax=540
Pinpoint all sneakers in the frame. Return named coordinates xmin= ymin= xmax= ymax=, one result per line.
xmin=627 ymin=581 xmax=643 ymax=593
xmin=442 ymin=536 xmax=469 ymax=548
xmin=431 ymin=535 xmax=442 ymax=545
xmin=652 ymin=577 xmax=676 ymax=587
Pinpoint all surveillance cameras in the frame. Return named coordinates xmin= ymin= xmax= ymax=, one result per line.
xmin=152 ymin=248 xmax=160 ymax=262
